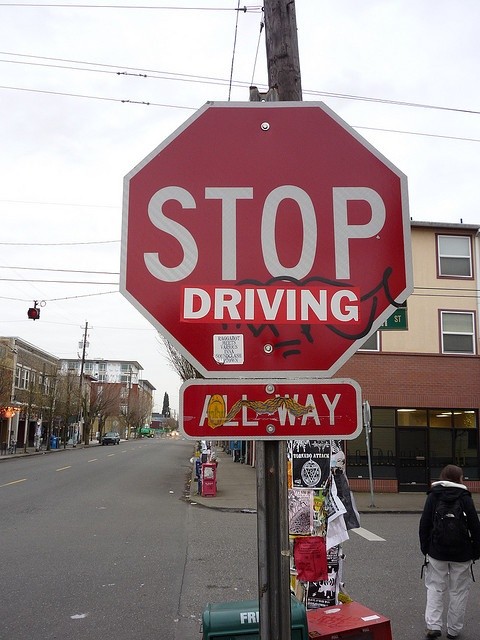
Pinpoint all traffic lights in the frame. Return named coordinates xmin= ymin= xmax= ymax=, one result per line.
xmin=26 ymin=307 xmax=41 ymax=321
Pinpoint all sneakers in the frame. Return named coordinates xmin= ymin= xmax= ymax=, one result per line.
xmin=424 ymin=629 xmax=442 ymax=639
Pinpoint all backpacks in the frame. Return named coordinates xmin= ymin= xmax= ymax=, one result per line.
xmin=419 ymin=491 xmax=477 ymax=584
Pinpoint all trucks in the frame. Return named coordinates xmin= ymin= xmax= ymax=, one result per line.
xmin=136 ymin=427 xmax=155 ymax=438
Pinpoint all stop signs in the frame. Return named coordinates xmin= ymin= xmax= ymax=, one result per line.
xmin=118 ymin=100 xmax=416 ymax=380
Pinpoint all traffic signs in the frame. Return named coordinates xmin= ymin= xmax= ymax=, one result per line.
xmin=178 ymin=379 xmax=362 ymax=440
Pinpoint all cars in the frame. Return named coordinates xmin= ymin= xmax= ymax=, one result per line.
xmin=100 ymin=431 xmax=120 ymax=445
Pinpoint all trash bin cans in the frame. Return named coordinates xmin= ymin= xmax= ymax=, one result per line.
xmin=56 ymin=437 xmax=62 ymax=448
xmin=50 ymin=436 xmax=57 ymax=449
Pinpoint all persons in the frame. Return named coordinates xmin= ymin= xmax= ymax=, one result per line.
xmin=10 ymin=430 xmax=17 ymax=454
xmin=419 ymin=464 xmax=479 ymax=640
